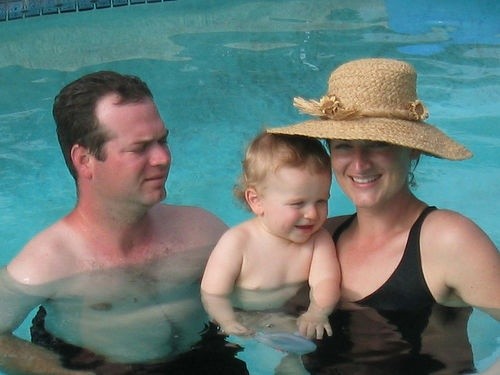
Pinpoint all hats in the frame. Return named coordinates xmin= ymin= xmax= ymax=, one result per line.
xmin=267 ymin=58 xmax=474 ymax=161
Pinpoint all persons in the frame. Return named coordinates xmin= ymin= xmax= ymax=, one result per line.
xmin=0 ymin=71 xmax=230 ymax=374
xmin=200 ymin=129 xmax=342 ymax=353
xmin=267 ymin=69 xmax=496 ymax=375
